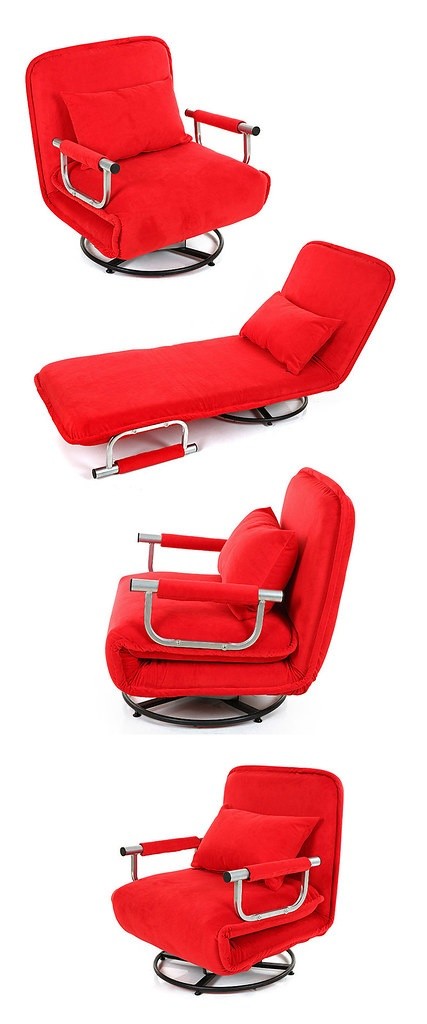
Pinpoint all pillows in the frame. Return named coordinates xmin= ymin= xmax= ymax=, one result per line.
xmin=218 ymin=506 xmax=300 ymax=622
xmin=238 ymin=291 xmax=343 ymax=375
xmin=191 ymin=802 xmax=322 ymax=890
xmin=59 ymin=74 xmax=193 ymax=169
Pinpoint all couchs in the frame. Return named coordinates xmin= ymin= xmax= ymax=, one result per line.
xmin=106 ymin=467 xmax=355 ymax=731
xmin=110 ymin=766 xmax=346 ymax=995
xmin=33 ymin=241 xmax=396 ymax=478
xmin=26 ymin=36 xmax=272 ymax=277
